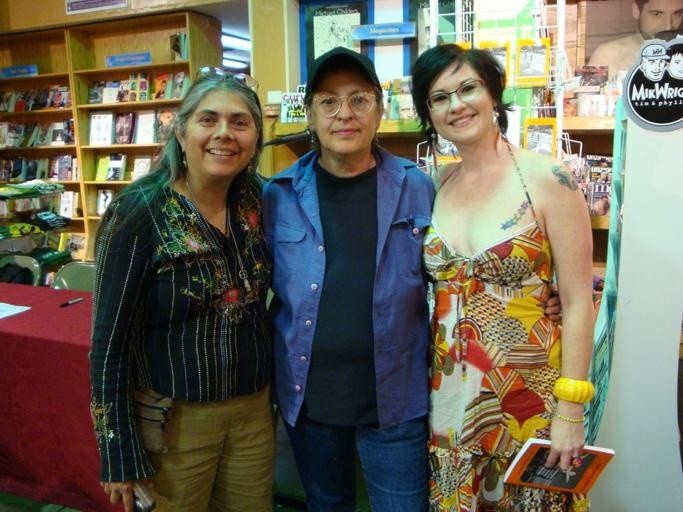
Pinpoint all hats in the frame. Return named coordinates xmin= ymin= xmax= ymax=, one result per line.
xmin=303 ymin=46 xmax=383 ymax=92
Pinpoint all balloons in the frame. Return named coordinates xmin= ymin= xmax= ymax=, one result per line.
xmin=0 ymin=67 xmax=185 ymax=268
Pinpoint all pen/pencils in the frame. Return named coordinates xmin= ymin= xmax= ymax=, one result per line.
xmin=59 ymin=297 xmax=83 ymax=308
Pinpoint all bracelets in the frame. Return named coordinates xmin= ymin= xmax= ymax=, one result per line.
xmin=555 ymin=412 xmax=585 ymax=423
xmin=553 ymin=377 xmax=596 ymax=406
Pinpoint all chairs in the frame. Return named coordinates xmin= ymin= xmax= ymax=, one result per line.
xmin=0 ymin=255 xmax=97 ymax=292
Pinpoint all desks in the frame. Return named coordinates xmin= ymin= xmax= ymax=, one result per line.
xmin=0 ymin=283 xmax=137 ymax=510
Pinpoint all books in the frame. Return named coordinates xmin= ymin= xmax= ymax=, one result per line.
xmin=571 ymin=65 xmax=625 ymax=218
xmin=174 ymin=33 xmax=189 ymax=62
xmin=530 ymin=82 xmax=578 ymax=119
xmin=501 ymin=437 xmax=615 ymax=496
xmin=478 ymin=41 xmax=512 ymax=87
xmin=430 ymin=134 xmax=462 ymax=166
xmin=521 ymin=117 xmax=557 ymax=158
xmin=168 ymin=32 xmax=184 ymax=62
xmin=454 ymin=40 xmax=473 ymax=52
xmin=279 ymin=75 xmax=426 ymax=124
xmin=513 ymin=39 xmax=549 ymax=89
xmin=493 ymin=104 xmax=521 ymax=148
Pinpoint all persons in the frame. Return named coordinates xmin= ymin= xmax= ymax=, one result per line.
xmin=585 ymin=1 xmax=682 ymax=86
xmin=639 ymin=43 xmax=671 ymax=83
xmin=412 ymin=41 xmax=599 ymax=510
xmin=664 ymin=43 xmax=682 ymax=82
xmin=233 ymin=43 xmax=567 ymax=511
xmin=171 ymin=44 xmax=182 ymax=62
xmin=85 ymin=74 xmax=279 ymax=512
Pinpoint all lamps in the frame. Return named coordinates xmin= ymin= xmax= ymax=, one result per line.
xmin=220 ymin=32 xmax=250 ymax=52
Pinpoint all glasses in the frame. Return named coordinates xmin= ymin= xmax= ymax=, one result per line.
xmin=312 ymin=89 xmax=376 ymax=118
xmin=192 ymin=66 xmax=261 ymax=96
xmin=426 ymin=77 xmax=485 ymax=113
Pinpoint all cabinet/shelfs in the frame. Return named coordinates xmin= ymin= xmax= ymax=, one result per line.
xmin=0 ymin=11 xmax=223 ymax=263
xmin=257 ymin=0 xmax=682 ymax=512
xmin=563 ymin=117 xmax=615 ymax=229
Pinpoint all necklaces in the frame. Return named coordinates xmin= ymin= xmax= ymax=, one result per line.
xmin=181 ymin=176 xmax=253 ymax=296
